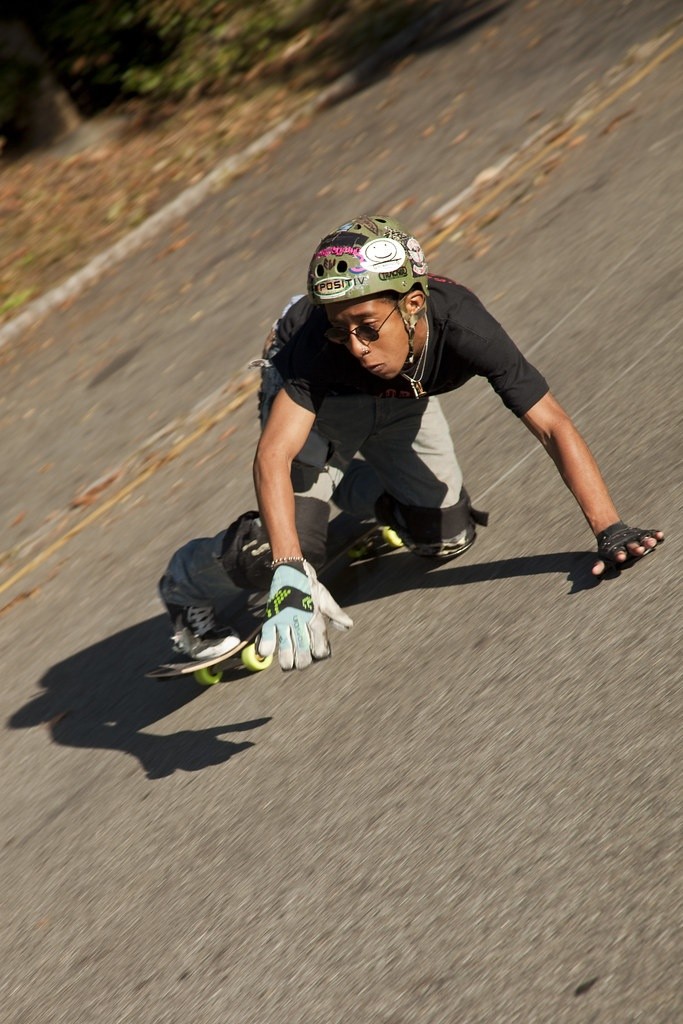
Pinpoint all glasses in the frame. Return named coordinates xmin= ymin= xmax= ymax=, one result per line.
xmin=325 ymin=295 xmax=401 ymax=345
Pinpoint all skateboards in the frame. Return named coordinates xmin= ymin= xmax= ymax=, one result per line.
xmin=141 ymin=508 xmax=402 ymax=687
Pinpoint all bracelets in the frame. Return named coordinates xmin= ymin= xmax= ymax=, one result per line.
xmin=271 ymin=557 xmax=304 ymax=570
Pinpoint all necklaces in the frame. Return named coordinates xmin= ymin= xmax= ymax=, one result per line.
xmin=400 ymin=313 xmax=429 ymax=400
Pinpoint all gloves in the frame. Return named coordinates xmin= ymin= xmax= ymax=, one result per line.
xmin=259 ymin=560 xmax=354 ymax=670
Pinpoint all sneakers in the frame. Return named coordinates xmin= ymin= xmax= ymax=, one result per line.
xmin=159 ymin=577 xmax=240 ymax=662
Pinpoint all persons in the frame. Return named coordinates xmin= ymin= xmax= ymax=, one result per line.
xmin=157 ymin=214 xmax=665 ymax=670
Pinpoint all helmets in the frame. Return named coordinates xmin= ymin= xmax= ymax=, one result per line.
xmin=307 ymin=215 xmax=430 ymax=305
